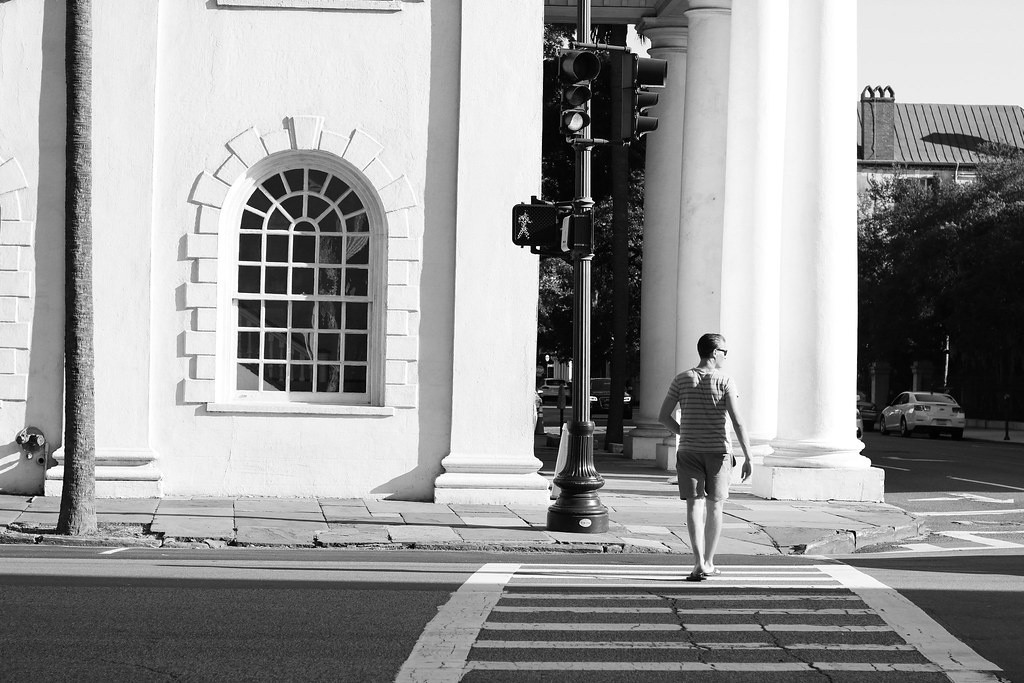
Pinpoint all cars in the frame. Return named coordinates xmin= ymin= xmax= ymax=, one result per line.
xmin=590 ymin=377 xmax=635 ymax=420
xmin=878 ymin=389 xmax=966 ymax=439
xmin=537 ymin=378 xmax=572 ymax=406
xmin=857 ymin=391 xmax=880 ymax=444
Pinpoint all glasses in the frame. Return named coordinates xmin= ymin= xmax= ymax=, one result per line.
xmin=710 ymin=349 xmax=727 ymax=356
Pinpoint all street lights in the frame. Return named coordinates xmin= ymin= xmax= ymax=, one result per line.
xmin=544 ymin=354 xmax=551 ymax=378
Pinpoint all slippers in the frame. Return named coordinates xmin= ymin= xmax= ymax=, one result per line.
xmin=686 ymin=573 xmax=702 ymax=581
xmin=704 ymin=567 xmax=721 ymax=576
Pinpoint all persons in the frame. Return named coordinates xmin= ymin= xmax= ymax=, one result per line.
xmin=657 ymin=333 xmax=753 ymax=582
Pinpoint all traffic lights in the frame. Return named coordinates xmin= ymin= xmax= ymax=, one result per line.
xmin=557 ymin=47 xmax=603 ymax=138
xmin=560 ymin=212 xmax=591 ymax=254
xmin=510 ymin=200 xmax=557 ymax=247
xmin=619 ymin=49 xmax=670 ymax=144
